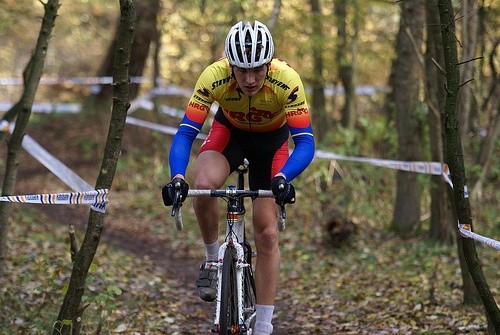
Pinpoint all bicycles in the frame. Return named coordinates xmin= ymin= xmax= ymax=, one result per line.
xmin=169 ymin=157 xmax=293 ymax=335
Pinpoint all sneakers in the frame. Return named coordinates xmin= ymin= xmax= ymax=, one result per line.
xmin=194 ymin=262 xmax=219 ymax=301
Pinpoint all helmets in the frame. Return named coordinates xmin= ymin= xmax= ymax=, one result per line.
xmin=224 ymin=18 xmax=276 ymax=70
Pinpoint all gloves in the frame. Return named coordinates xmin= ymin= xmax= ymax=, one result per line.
xmin=160 ymin=176 xmax=188 ymax=210
xmin=269 ymin=176 xmax=300 ymax=209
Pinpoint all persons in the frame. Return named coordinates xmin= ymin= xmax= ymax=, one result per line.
xmin=161 ymin=18 xmax=316 ymax=335
